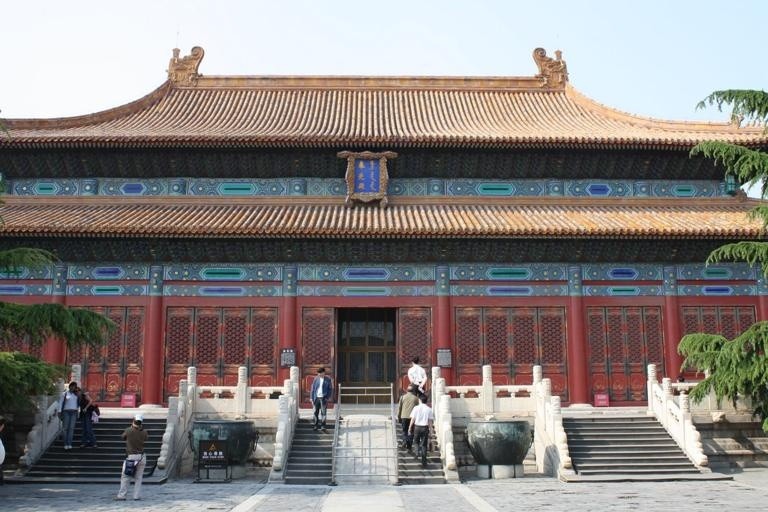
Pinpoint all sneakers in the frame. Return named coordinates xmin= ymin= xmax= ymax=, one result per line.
xmin=63 ymin=443 xmax=98 ymax=450
xmin=313 ymin=424 xmax=326 ymax=431
xmin=112 ymin=496 xmax=126 ymax=501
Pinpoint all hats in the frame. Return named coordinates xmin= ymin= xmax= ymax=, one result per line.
xmin=135 ymin=415 xmax=144 ymax=422
xmin=317 ymin=368 xmax=325 ymax=373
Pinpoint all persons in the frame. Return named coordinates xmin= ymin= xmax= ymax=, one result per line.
xmin=397 ymin=384 xmax=419 ymax=450
xmin=309 ymin=367 xmax=332 ymax=431
xmin=58 ymin=382 xmax=89 ymax=450
xmin=0 ymin=424 xmax=6 ymax=466
xmin=407 ymin=395 xmax=434 ymax=466
xmin=673 ymin=376 xmax=689 ymax=395
xmin=112 ymin=415 xmax=148 ymax=501
xmin=407 ymin=358 xmax=427 ymax=396
xmin=74 ymin=387 xmax=97 ymax=448
xmin=91 ymin=403 xmax=100 ymax=423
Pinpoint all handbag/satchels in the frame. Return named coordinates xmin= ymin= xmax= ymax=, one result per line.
xmin=90 ymin=405 xmax=101 ymax=417
xmin=124 ymin=459 xmax=138 ymax=476
